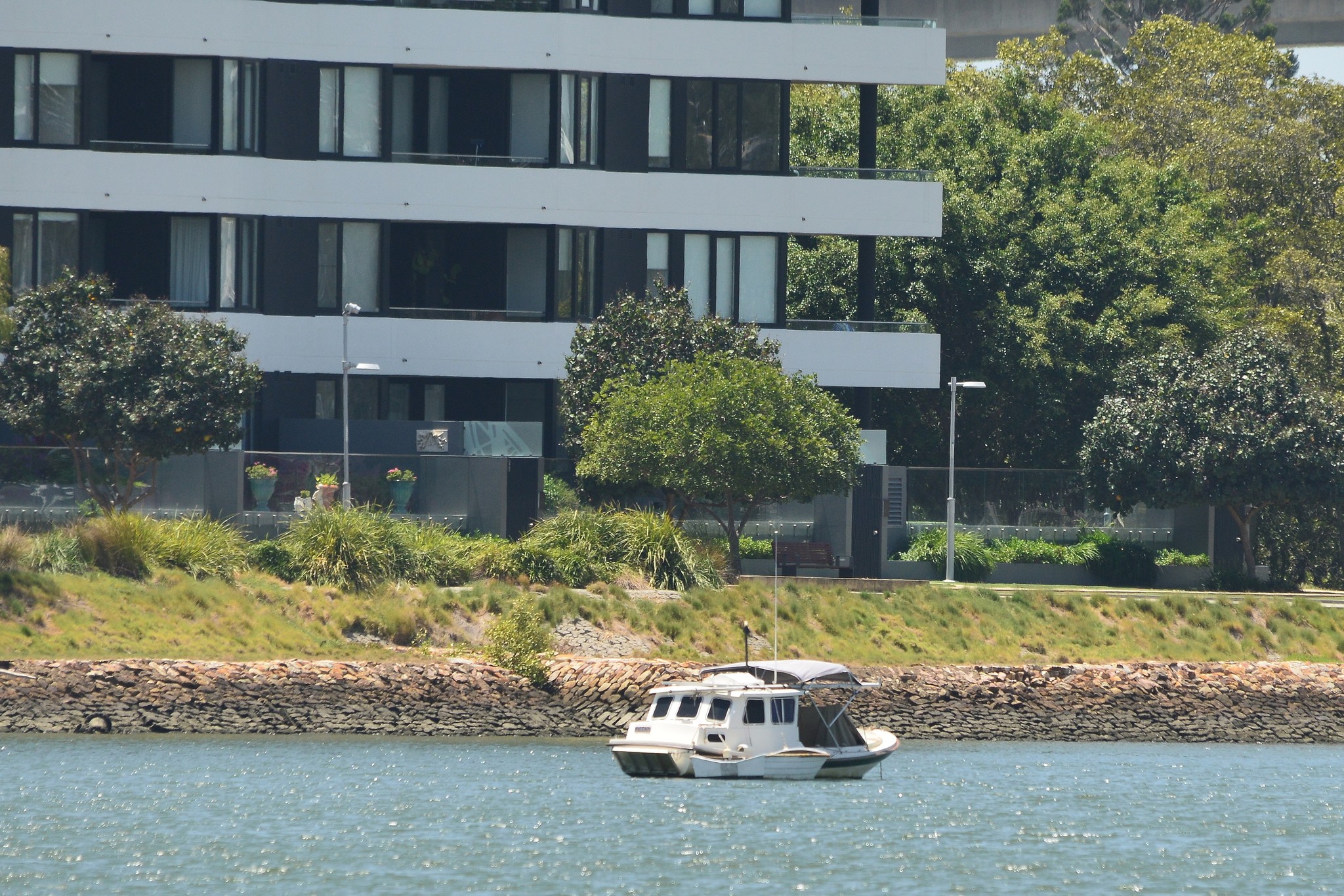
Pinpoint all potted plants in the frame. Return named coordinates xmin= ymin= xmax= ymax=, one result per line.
xmin=314 ymin=472 xmax=339 ymax=511
xmin=294 ymin=490 xmax=312 ymax=513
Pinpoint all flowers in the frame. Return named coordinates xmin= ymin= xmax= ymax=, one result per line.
xmin=245 ymin=462 xmax=278 ymax=477
xmin=386 ymin=468 xmax=418 ymax=482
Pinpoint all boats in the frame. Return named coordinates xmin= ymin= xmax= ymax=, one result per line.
xmin=604 ymin=530 xmax=900 ymax=780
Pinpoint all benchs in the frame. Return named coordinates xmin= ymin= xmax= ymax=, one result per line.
xmin=771 ymin=541 xmax=850 ymax=577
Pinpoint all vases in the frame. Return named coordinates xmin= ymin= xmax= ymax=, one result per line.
xmin=388 ymin=480 xmax=415 ymax=513
xmin=249 ymin=476 xmax=277 ymax=511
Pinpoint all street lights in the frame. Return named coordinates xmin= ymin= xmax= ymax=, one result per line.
xmin=943 ymin=376 xmax=987 ymax=582
xmin=340 ymin=303 xmax=381 ymax=513
